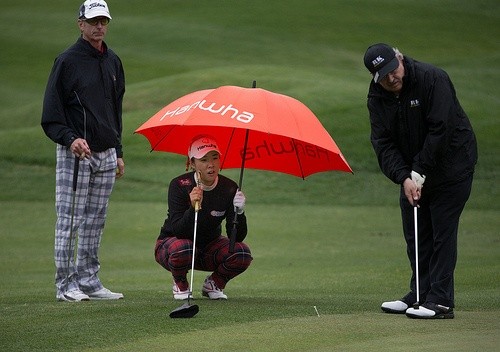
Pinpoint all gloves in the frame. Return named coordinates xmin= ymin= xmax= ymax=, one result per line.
xmin=411 ymin=170 xmax=426 ymax=193
xmin=233 ymin=188 xmax=246 ymax=214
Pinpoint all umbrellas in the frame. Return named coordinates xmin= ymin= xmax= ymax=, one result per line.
xmin=131 ymin=81 xmax=354 ymax=255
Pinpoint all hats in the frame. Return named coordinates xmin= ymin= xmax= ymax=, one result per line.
xmin=364 ymin=43 xmax=399 ymax=83
xmin=188 ymin=134 xmax=222 ymax=160
xmin=78 ymin=0 xmax=112 ymax=20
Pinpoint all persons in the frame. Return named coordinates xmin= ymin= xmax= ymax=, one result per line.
xmin=39 ymin=1 xmax=127 ymax=302
xmin=151 ymin=137 xmax=252 ymax=300
xmin=361 ymin=45 xmax=478 ymax=321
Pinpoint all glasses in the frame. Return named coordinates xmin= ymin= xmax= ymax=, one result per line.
xmin=380 ymin=69 xmax=396 ymax=81
xmin=81 ymin=17 xmax=109 ymax=26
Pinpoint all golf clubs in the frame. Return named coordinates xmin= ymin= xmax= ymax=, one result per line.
xmin=413 ymin=199 xmax=420 ymax=310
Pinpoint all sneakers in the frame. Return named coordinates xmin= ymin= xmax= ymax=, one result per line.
xmin=172 ymin=274 xmax=194 ymax=300
xmin=84 ymin=286 xmax=124 ymax=300
xmin=381 ymin=291 xmax=426 ymax=313
xmin=406 ymin=300 xmax=454 ymax=319
xmin=201 ymin=275 xmax=228 ymax=300
xmin=56 ymin=288 xmax=90 ymax=302
